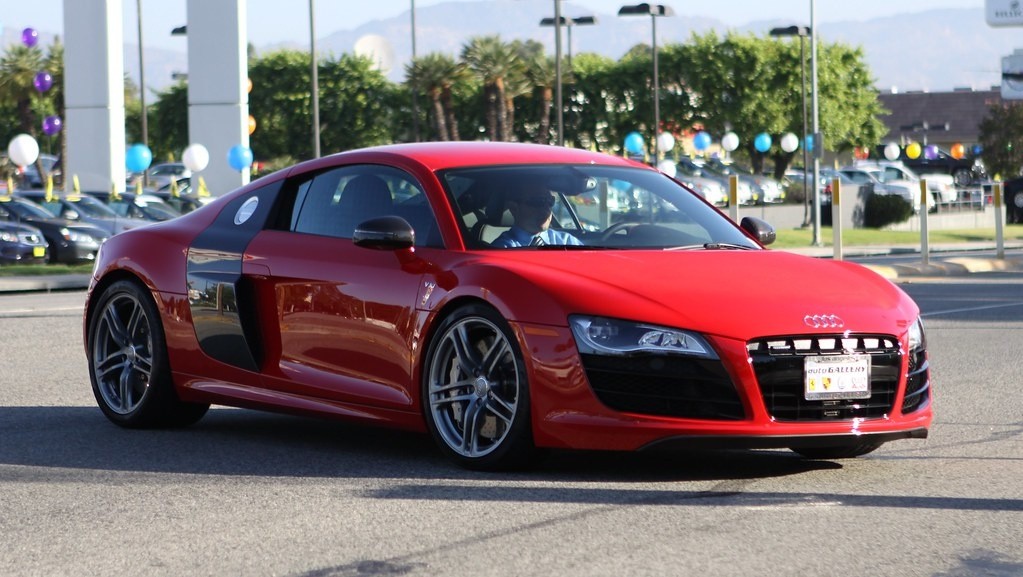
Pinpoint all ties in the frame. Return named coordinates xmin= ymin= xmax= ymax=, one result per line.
xmin=533 ymin=237 xmax=544 ymax=247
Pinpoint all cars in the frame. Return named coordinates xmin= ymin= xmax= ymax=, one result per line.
xmin=0 ymin=151 xmax=216 ymax=266
xmin=590 ymin=156 xmax=991 ymax=217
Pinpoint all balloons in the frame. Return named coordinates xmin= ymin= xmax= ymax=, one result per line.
xmin=659 ymin=132 xmax=674 ymax=152
xmin=722 ymin=132 xmax=738 ymax=151
xmin=973 ymin=144 xmax=983 ymax=155
xmin=694 ymin=132 xmax=711 ymax=150
xmin=22 ymin=27 xmax=39 ymax=48
xmin=923 ymin=143 xmax=938 ymax=161
xmin=127 ymin=144 xmax=152 ymax=172
xmin=782 ymin=132 xmax=799 ymax=152
xmin=755 ymin=132 xmax=771 ymax=152
xmin=626 ymin=133 xmax=644 ymax=154
xmin=34 ymin=71 xmax=51 ymax=90
xmin=905 ymin=141 xmax=921 ymax=160
xmin=856 ymin=147 xmax=869 ymax=159
xmin=181 ymin=78 xmax=256 ymax=173
xmin=7 ymin=134 xmax=38 ymax=167
xmin=884 ymin=141 xmax=900 ymax=162
xmin=802 ymin=134 xmax=814 ymax=152
xmin=951 ymin=143 xmax=964 ymax=159
xmin=43 ymin=115 xmax=61 ymax=134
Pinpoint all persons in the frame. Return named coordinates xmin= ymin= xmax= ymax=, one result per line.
xmin=488 ymin=177 xmax=585 ymax=250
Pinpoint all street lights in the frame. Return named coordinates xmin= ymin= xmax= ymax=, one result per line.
xmin=771 ymin=25 xmax=812 ymax=228
xmin=542 ymin=15 xmax=597 ymax=64
xmin=619 ymin=3 xmax=673 ymax=167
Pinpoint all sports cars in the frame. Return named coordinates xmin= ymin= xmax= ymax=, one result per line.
xmin=82 ymin=142 xmax=934 ymax=473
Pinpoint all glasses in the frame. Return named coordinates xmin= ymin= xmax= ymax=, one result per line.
xmin=516 ymin=196 xmax=555 ymax=208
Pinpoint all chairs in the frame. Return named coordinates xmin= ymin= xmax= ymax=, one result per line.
xmin=472 ymin=189 xmax=516 ymax=246
xmin=335 ymin=171 xmax=395 ymax=229
